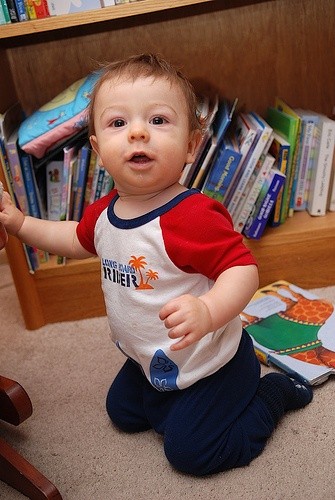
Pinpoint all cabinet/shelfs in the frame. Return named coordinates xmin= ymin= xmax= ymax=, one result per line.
xmin=0 ymin=0 xmax=335 ymax=330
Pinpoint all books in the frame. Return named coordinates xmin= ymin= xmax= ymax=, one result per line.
xmin=0 ymin=0 xmax=139 ymax=24
xmin=0 ymin=96 xmax=335 ymax=389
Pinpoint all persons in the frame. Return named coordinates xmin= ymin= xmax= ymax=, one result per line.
xmin=0 ymin=54 xmax=313 ymax=478
